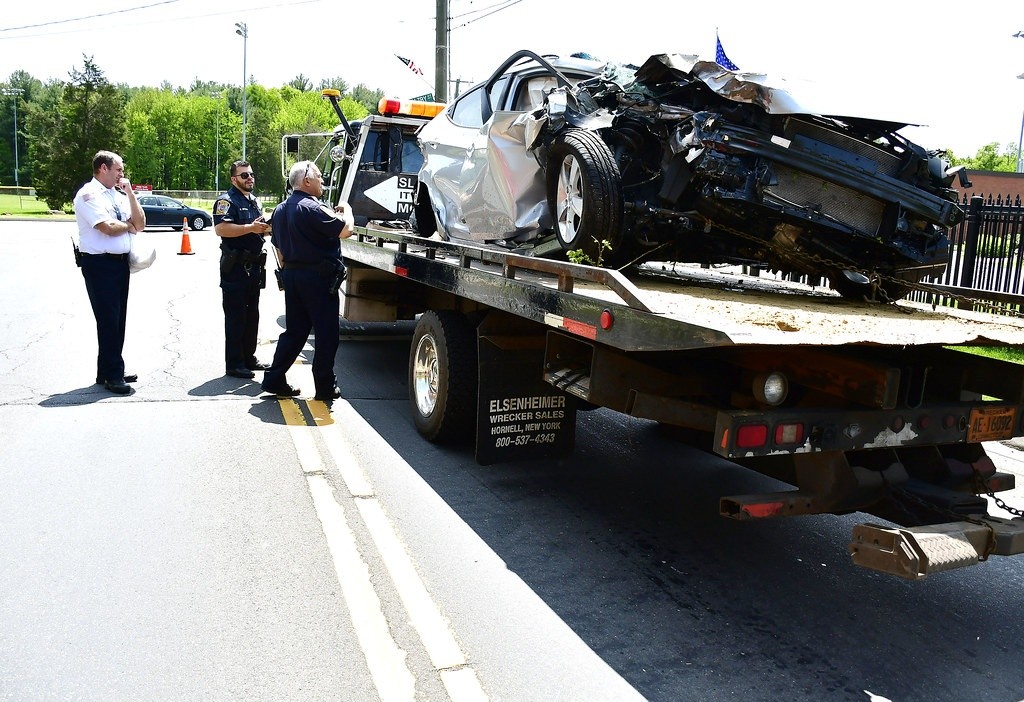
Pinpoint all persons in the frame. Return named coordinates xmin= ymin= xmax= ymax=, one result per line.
xmin=74 ymin=150 xmax=148 ymax=395
xmin=262 ymin=160 xmax=355 ymax=400
xmin=213 ymin=161 xmax=272 ymax=377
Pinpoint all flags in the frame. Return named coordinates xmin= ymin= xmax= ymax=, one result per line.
xmin=715 ymin=35 xmax=738 ymax=71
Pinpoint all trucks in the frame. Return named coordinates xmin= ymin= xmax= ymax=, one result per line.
xmin=277 ymin=84 xmax=1024 ymax=576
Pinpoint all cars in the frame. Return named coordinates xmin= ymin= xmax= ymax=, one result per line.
xmin=136 ymin=195 xmax=213 ymax=230
xmin=410 ymin=31 xmax=972 ymax=305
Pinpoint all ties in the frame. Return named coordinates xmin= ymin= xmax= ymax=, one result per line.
xmin=106 ymin=190 xmax=122 ymax=221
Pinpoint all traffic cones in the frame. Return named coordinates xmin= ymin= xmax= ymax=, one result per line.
xmin=176 ymin=216 xmax=195 ymax=255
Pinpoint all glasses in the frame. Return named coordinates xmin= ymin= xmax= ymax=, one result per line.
xmin=234 ymin=172 xmax=256 ymax=179
xmin=303 ymin=160 xmax=312 ymax=179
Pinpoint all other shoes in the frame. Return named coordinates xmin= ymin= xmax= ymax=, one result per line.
xmin=260 ymin=381 xmax=301 ymax=396
xmin=314 ymin=385 xmax=341 ymax=400
xmin=96 ymin=370 xmax=137 ymax=394
xmin=226 ymin=368 xmax=255 ymax=379
xmin=246 ymin=358 xmax=272 ymax=370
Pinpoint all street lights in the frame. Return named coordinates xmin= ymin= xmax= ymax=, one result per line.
xmin=233 ymin=20 xmax=249 ymax=164
xmin=209 ymin=92 xmax=225 ymax=198
xmin=2 ymin=87 xmax=26 ymax=196
